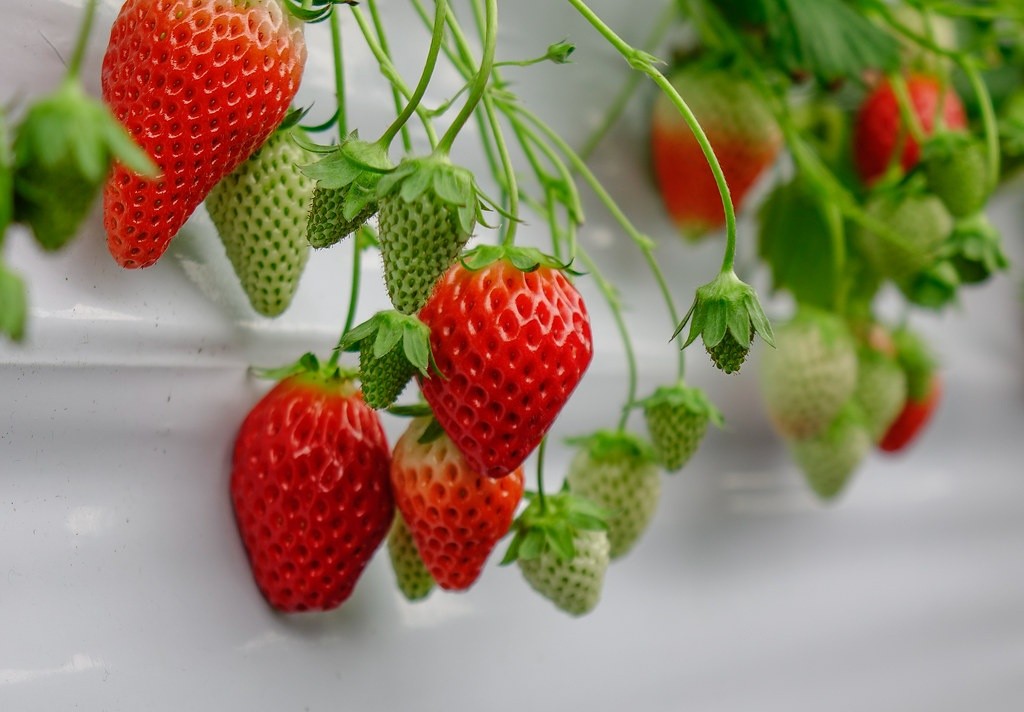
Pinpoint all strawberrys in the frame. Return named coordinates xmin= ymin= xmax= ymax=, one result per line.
xmin=0 ymin=0 xmax=986 ymax=614
